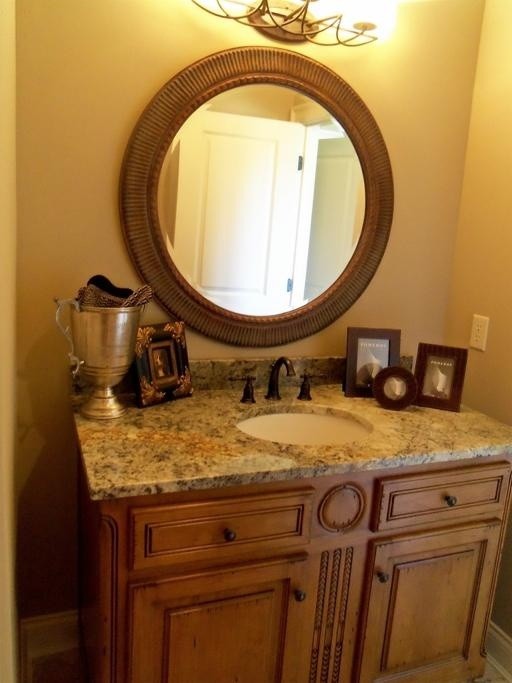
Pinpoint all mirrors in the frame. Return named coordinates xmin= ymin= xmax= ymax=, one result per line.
xmin=118 ymin=43 xmax=397 ymax=346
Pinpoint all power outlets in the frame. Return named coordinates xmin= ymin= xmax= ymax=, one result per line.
xmin=469 ymin=314 xmax=490 ymax=351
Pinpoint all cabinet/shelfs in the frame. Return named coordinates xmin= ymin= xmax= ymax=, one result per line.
xmin=76 ymin=447 xmax=333 ymax=683
xmin=335 ymin=455 xmax=511 ymax=683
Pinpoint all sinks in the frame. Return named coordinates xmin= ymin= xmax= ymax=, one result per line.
xmin=228 ymin=397 xmax=377 ymax=450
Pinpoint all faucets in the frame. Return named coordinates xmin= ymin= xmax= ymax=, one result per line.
xmin=265 ymin=353 xmax=296 ymax=401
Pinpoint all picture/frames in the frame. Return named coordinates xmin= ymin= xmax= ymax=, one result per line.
xmin=133 ymin=320 xmax=195 ymax=406
xmin=343 ymin=328 xmax=468 ymax=413
